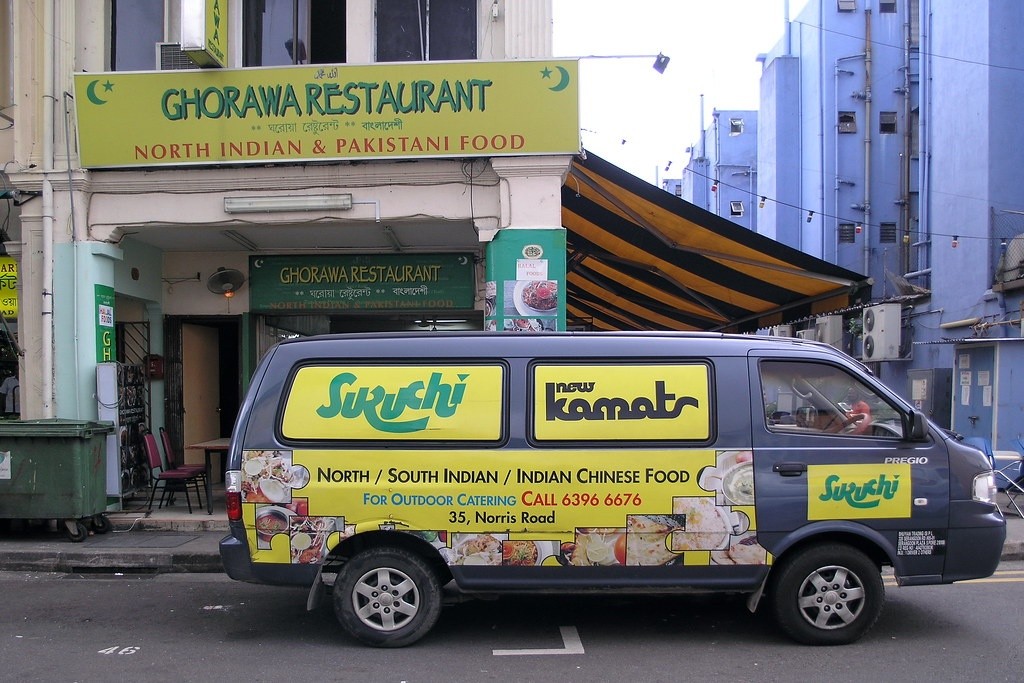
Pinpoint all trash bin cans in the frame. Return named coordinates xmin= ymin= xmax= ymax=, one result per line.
xmin=0 ymin=418 xmax=116 ymax=519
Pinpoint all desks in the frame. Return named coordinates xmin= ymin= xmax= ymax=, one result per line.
xmin=185 ymin=437 xmax=232 ymax=515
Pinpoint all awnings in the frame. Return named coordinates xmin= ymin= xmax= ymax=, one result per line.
xmin=562 ymin=148 xmax=873 ymax=333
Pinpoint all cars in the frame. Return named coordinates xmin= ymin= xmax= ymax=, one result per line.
xmin=765 ymin=417 xmax=1024 ymax=493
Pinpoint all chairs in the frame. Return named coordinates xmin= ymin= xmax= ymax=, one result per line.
xmin=960 ymin=437 xmax=1007 ymax=526
xmin=138 ymin=422 xmax=203 ymax=514
xmin=159 ymin=427 xmax=212 ymax=506
xmin=995 ymin=433 xmax=1024 ymax=508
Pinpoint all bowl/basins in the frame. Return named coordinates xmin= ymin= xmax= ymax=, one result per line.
xmin=674 ymin=497 xmax=733 ymax=550
xmin=521 ymin=280 xmax=557 ymax=311
xmin=532 ymin=540 xmax=553 ymax=566
xmin=522 ymin=245 xmax=543 ymax=259
xmin=721 ymin=461 xmax=755 ymax=505
xmin=254 ymin=510 xmax=287 ymax=542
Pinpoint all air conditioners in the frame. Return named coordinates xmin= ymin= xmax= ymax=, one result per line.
xmin=768 ymin=325 xmax=791 ymax=337
xmin=155 ymin=42 xmax=201 ymax=70
xmin=796 ymin=329 xmax=815 ymax=340
xmin=815 ymin=315 xmax=842 ymax=351
xmin=863 ymin=303 xmax=902 ymax=360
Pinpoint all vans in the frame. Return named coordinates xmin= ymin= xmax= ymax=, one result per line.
xmin=219 ymin=329 xmax=1007 ymax=648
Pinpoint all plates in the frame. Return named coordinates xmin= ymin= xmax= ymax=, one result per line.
xmin=513 ymin=280 xmax=558 ymax=316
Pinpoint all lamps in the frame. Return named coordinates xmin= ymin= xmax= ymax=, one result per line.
xmin=283 ymin=37 xmax=308 ymax=65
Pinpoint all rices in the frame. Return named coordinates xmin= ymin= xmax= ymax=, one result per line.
xmin=673 ymin=497 xmax=726 ymax=550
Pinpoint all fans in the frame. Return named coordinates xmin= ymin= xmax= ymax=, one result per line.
xmin=206 ymin=266 xmax=245 ymax=298
